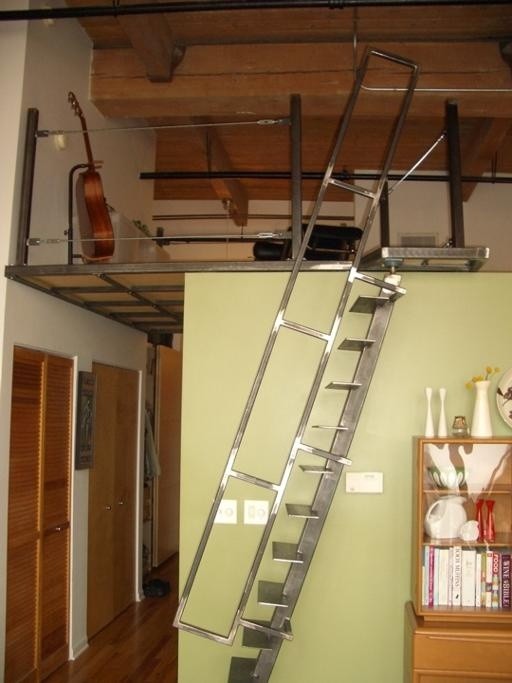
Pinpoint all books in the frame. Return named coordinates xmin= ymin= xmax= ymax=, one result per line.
xmin=422 ymin=546 xmax=512 ymax=609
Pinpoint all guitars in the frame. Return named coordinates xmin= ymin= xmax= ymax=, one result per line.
xmin=68 ymin=90 xmax=115 ymax=264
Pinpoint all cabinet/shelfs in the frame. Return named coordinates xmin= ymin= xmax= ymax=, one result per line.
xmin=401 ymin=433 xmax=512 ymax=682
